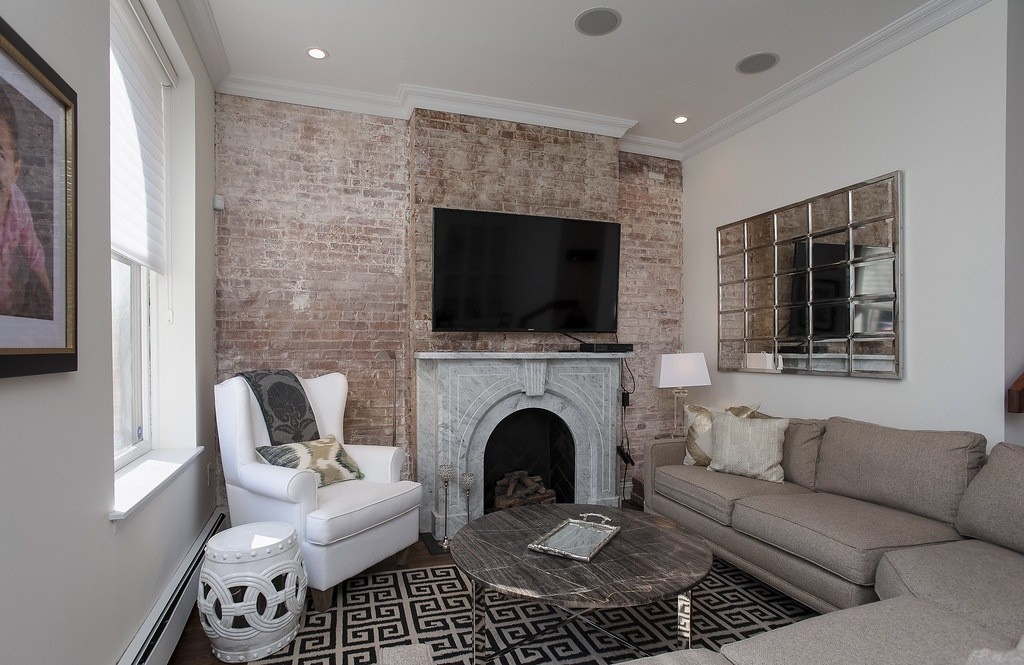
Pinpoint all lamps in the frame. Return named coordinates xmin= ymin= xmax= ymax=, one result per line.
xmin=652 ymin=349 xmax=712 ymax=437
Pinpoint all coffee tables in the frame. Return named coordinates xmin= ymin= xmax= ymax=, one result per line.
xmin=449 ymin=506 xmax=712 ymax=665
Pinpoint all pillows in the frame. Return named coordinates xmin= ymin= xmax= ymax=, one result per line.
xmin=255 ymin=433 xmax=364 ymax=493
xmin=705 ymin=414 xmax=795 ymax=485
xmin=679 ymin=402 xmax=762 ymax=469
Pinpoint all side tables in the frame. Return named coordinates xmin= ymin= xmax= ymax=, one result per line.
xmin=193 ymin=523 xmax=306 ymax=662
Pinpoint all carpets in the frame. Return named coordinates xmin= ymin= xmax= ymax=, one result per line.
xmin=243 ymin=556 xmax=813 ymax=665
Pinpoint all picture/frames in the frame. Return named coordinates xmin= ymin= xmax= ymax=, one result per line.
xmin=0 ymin=16 xmax=77 ymax=377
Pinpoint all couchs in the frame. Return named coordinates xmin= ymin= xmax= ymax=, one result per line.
xmin=585 ymin=412 xmax=1023 ymax=665
xmin=213 ymin=371 xmax=423 ymax=594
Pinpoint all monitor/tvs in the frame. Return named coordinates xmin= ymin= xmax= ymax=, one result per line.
xmin=432 ymin=206 xmax=622 ymax=333
